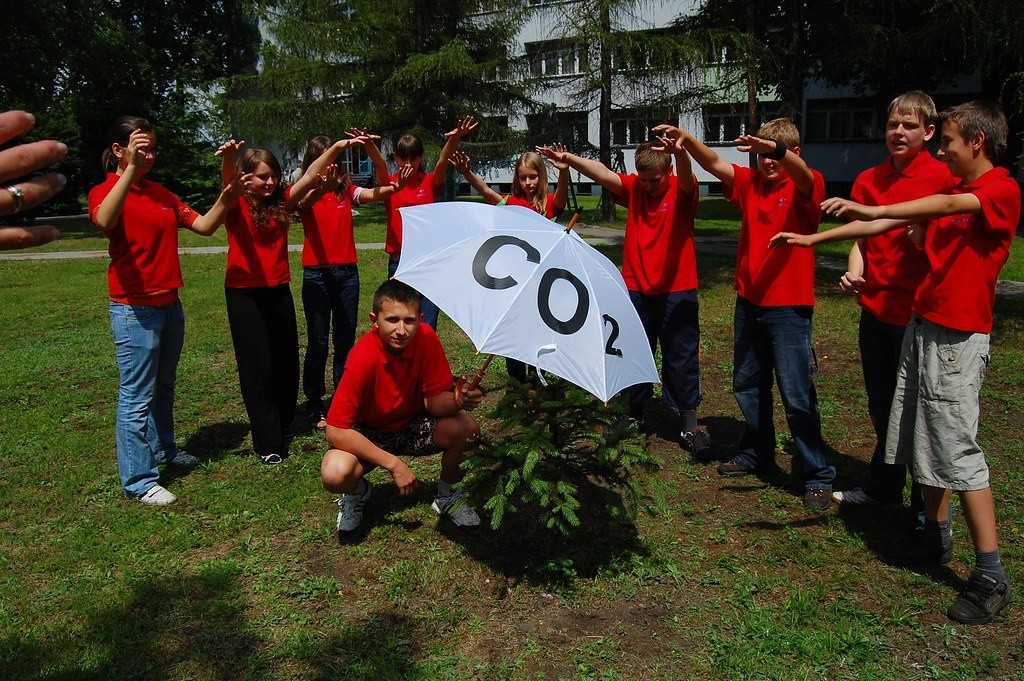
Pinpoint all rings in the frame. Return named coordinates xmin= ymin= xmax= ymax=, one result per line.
xmin=5 ymin=185 xmax=24 ymax=215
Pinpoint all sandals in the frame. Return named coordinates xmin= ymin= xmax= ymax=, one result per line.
xmin=308 ymin=409 xmax=328 ymax=430
xmin=259 ymin=452 xmax=282 ymax=465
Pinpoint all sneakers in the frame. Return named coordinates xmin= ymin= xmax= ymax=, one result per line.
xmin=172 ymin=448 xmax=199 ymax=465
xmin=947 ymin=569 xmax=1011 ymax=624
xmin=133 ymin=484 xmax=176 ymax=506
xmin=678 ymin=428 xmax=712 ymax=454
xmin=879 ymin=531 xmax=954 ymax=565
xmin=431 ymin=490 xmax=481 ymax=529
xmin=718 ymin=458 xmax=758 ymax=475
xmin=834 ymin=486 xmax=905 ymax=513
xmin=607 ymin=415 xmax=647 ymax=440
xmin=334 ymin=480 xmax=373 ymax=536
xmin=804 ymin=486 xmax=832 ymax=510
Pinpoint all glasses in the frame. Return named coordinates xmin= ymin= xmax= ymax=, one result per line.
xmin=138 ymin=147 xmax=162 ymax=156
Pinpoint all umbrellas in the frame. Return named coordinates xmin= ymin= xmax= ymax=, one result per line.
xmin=390 ymin=202 xmax=663 ymax=408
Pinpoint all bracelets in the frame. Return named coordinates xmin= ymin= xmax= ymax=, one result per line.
xmin=761 ymin=138 xmax=787 ymax=160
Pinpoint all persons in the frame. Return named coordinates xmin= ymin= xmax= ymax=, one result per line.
xmin=213 ymin=136 xmax=369 ymax=464
xmin=88 ymin=116 xmax=253 ymax=505
xmin=534 ymin=137 xmax=713 ymax=460
xmin=767 ymin=100 xmax=1021 ymax=625
xmin=292 ymin=135 xmax=413 ymax=431
xmin=345 ymin=115 xmax=478 ymax=333
xmin=832 ymin=91 xmax=963 ymax=537
xmin=652 ymin=119 xmax=836 ymax=510
xmin=448 ymin=142 xmax=570 ymax=387
xmin=0 ymin=110 xmax=68 ymax=250
xmin=321 ymin=279 xmax=482 ymax=532
